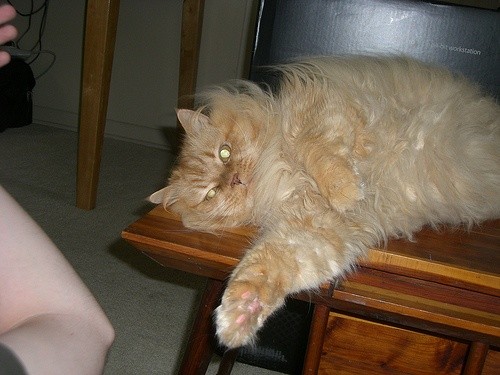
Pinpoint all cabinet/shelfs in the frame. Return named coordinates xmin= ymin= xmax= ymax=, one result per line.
xmin=121 ymin=198 xmax=500 ymax=375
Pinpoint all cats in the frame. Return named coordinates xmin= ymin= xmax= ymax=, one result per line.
xmin=147 ymin=47 xmax=500 ymax=354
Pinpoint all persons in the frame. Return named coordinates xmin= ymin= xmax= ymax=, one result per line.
xmin=1 ymin=4 xmax=114 ymax=375
